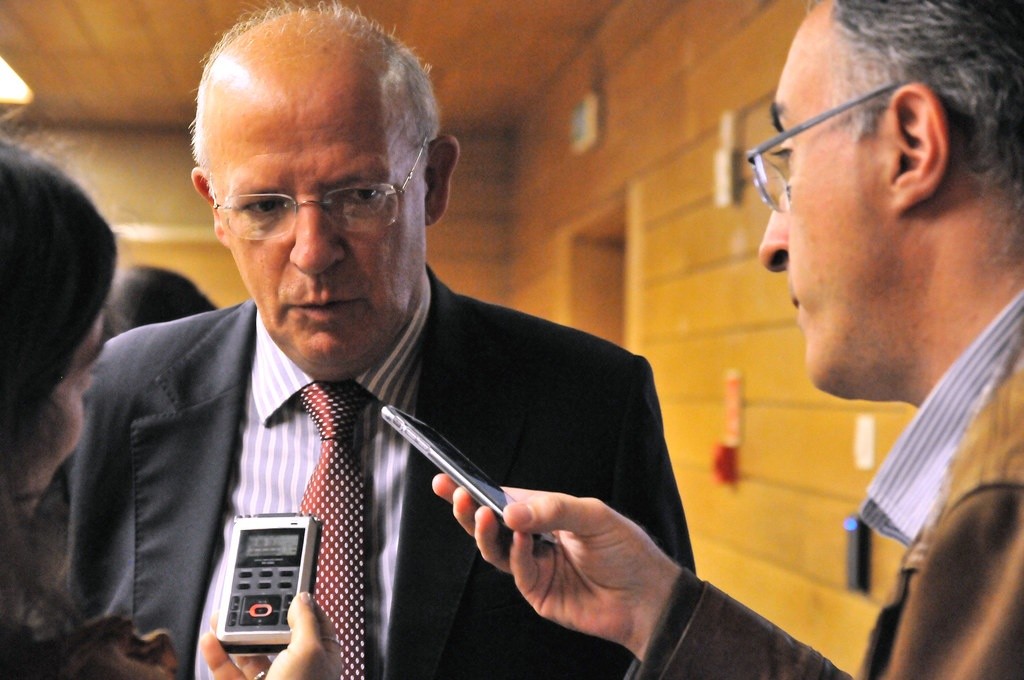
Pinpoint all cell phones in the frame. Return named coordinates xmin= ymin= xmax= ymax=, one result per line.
xmin=380 ymin=406 xmax=559 ymax=548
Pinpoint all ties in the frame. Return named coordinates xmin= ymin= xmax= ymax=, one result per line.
xmin=295 ymin=382 xmax=376 ymax=680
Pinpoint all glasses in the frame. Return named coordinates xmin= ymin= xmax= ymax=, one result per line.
xmin=746 ymin=78 xmax=904 ymax=213
xmin=209 ymin=134 xmax=429 ymax=237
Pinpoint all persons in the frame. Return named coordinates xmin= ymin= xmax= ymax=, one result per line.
xmin=18 ymin=8 xmax=695 ymax=680
xmin=0 ymin=142 xmax=341 ymax=680
xmin=103 ymin=266 xmax=217 ymax=343
xmin=431 ymin=0 xmax=1024 ymax=680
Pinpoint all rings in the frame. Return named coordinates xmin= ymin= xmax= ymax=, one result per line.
xmin=253 ymin=671 xmax=266 ymax=680
xmin=321 ymin=637 xmax=340 ymax=644
